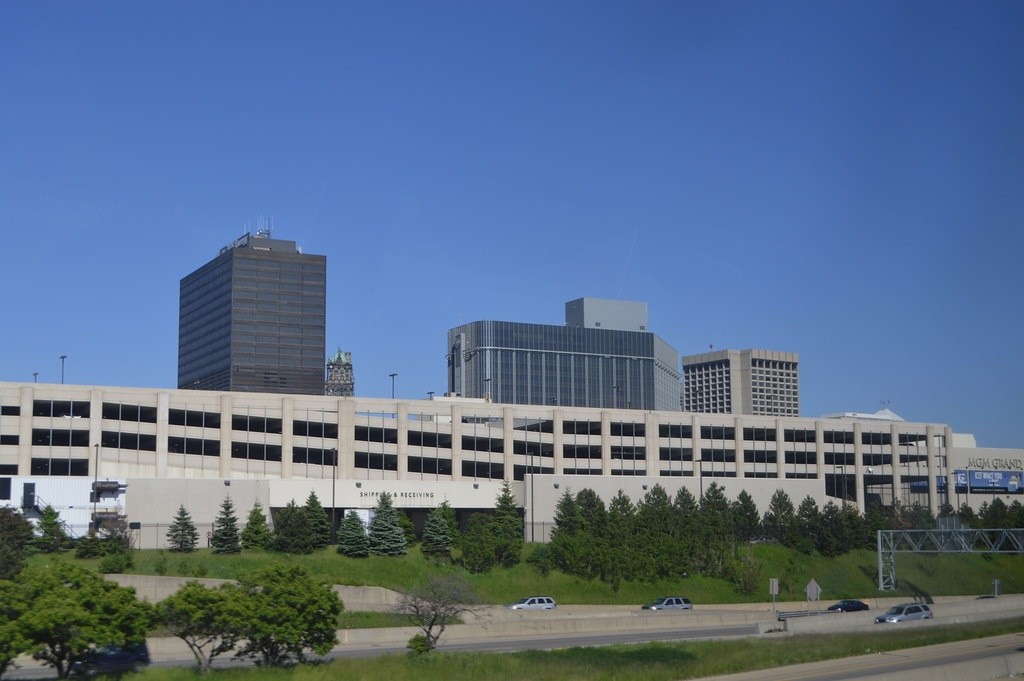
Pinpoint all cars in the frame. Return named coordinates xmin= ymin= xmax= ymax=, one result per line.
xmin=828 ymin=599 xmax=870 ymax=613
xmin=74 ymin=639 xmax=158 ymax=676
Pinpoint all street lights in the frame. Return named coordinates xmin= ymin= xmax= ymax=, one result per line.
xmin=33 ymin=373 xmax=39 ymax=382
xmin=388 ymin=373 xmax=398 ymax=418
xmin=427 ymin=392 xmax=435 ymax=421
xmin=60 ymin=355 xmax=67 ymax=384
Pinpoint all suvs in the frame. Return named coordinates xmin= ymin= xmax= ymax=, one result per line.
xmin=874 ymin=604 xmax=933 ymax=624
xmin=642 ymin=597 xmax=692 ymax=611
xmin=507 ymin=596 xmax=557 ymax=610
